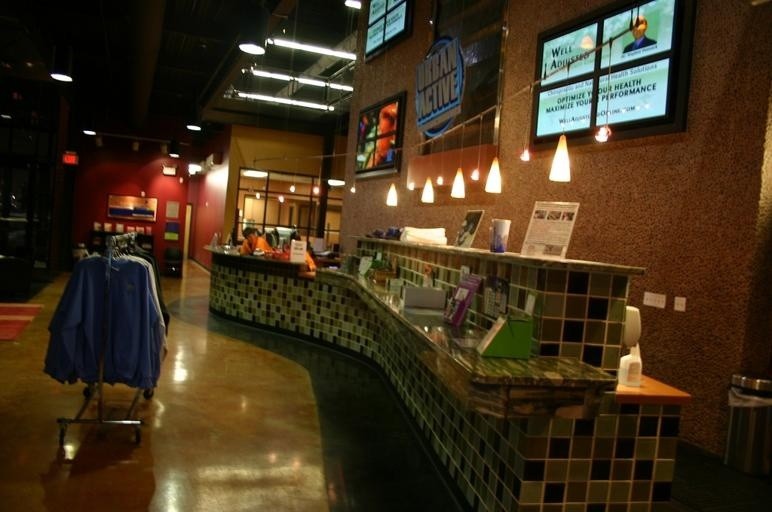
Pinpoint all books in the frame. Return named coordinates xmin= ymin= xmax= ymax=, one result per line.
xmin=441 ymin=272 xmax=485 ymax=325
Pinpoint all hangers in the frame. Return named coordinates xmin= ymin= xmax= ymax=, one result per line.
xmin=102 ymin=233 xmax=131 ymax=271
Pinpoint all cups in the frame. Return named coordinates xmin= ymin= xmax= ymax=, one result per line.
xmin=490 ymin=218 xmax=511 ymax=253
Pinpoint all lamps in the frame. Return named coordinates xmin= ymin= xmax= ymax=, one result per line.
xmin=49 ymin=39 xmax=76 ymax=83
xmin=168 ymin=2 xmax=269 ymax=159
xmin=384 ymin=21 xmax=644 ymax=208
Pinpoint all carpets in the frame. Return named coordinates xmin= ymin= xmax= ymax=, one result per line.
xmin=0 ymin=303 xmax=44 ymax=342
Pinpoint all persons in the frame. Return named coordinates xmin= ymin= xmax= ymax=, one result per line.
xmin=277 ymin=230 xmax=317 ymax=272
xmin=623 ymin=14 xmax=657 ymax=53
xmin=238 ymin=226 xmax=273 ymax=257
xmin=365 ymin=102 xmax=397 ymax=168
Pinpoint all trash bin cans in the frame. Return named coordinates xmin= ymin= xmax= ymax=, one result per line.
xmin=164 ymin=247 xmax=183 ymax=275
xmin=724 ymin=373 xmax=771 ymax=474
